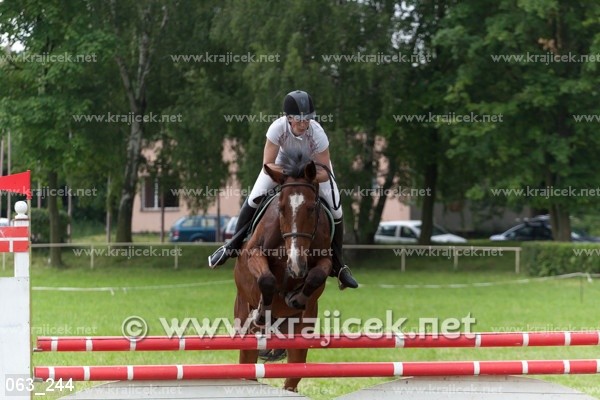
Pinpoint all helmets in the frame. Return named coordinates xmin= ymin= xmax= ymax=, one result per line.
xmin=284 ymin=90 xmax=316 ymax=121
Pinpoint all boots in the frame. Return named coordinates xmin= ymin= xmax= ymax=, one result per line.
xmin=329 ymin=215 xmax=358 ymax=288
xmin=211 ymin=199 xmax=258 ymax=266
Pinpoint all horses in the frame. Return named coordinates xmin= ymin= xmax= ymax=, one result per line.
xmin=234 ymin=162 xmax=334 ymax=393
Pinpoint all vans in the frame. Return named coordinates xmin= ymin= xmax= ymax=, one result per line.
xmin=170 ymin=214 xmax=230 ymax=241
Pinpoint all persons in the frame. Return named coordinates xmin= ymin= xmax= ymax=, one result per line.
xmin=210 ymin=89 xmax=359 ymax=290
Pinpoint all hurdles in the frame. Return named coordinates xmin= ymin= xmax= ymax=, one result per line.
xmin=0 ymin=200 xmax=598 ymax=400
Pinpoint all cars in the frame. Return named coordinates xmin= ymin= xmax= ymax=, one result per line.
xmin=489 ymin=218 xmax=582 ymax=242
xmin=372 ymin=219 xmax=467 ymax=245
